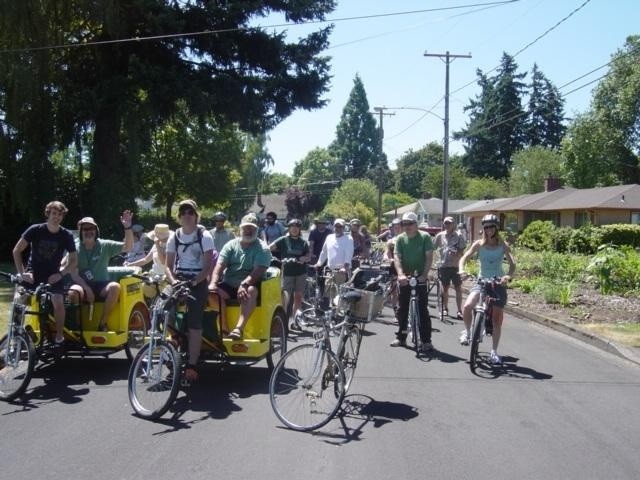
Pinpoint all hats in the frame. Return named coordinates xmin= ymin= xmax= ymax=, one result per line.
xmin=315 ymin=217 xmax=330 ymax=224
xmin=334 ymin=219 xmax=345 ymax=227
xmin=351 ymin=219 xmax=361 ymax=226
xmin=240 ymin=214 xmax=258 ymax=229
xmin=144 ymin=224 xmax=175 ymax=242
xmin=402 ymin=212 xmax=418 ymax=222
xmin=443 ymin=216 xmax=454 ymax=223
xmin=77 ymin=216 xmax=100 ymax=232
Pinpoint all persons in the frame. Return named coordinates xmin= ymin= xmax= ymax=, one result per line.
xmin=268 ymin=218 xmax=311 ymax=330
xmin=432 ymin=216 xmax=466 ymax=321
xmin=390 ymin=213 xmax=432 ymax=351
xmin=164 ymin=200 xmax=214 ymax=380
xmin=459 ymin=214 xmax=517 ymax=364
xmin=348 ymin=218 xmax=364 ymax=257
xmin=361 ymin=225 xmax=371 ymax=258
xmin=261 ymin=212 xmax=286 ymax=245
xmin=387 ymin=219 xmax=403 ymax=336
xmin=311 ymin=218 xmax=355 ymax=303
xmin=123 ymin=223 xmax=174 ymax=306
xmin=308 ymin=217 xmax=333 ymax=266
xmin=208 ymin=214 xmax=272 ymax=338
xmin=123 ymin=225 xmax=145 ymax=267
xmin=67 ymin=209 xmax=135 ymax=332
xmin=12 ymin=201 xmax=79 ymax=343
xmin=207 ymin=212 xmax=236 ymax=255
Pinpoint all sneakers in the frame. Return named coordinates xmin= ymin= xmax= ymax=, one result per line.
xmin=491 ymin=353 xmax=501 ymax=364
xmin=443 ymin=309 xmax=448 ymax=316
xmin=390 ymin=340 xmax=407 ymax=347
xmin=290 ymin=321 xmax=302 ymax=331
xmin=421 ymin=341 xmax=433 ymax=351
xmin=457 ymin=312 xmax=463 ymax=320
xmin=54 ymin=342 xmax=65 ymax=356
xmin=97 ymin=324 xmax=108 ymax=331
xmin=460 ymin=330 xmax=469 ymax=345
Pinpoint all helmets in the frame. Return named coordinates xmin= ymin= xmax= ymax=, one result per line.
xmin=214 ymin=212 xmax=226 ymax=220
xmin=391 ymin=218 xmax=402 ymax=225
xmin=287 ymin=218 xmax=302 ymax=237
xmin=131 ymin=225 xmax=144 ymax=239
xmin=360 ymin=225 xmax=367 ymax=230
xmin=481 ymin=213 xmax=501 ymax=230
xmin=389 ymin=223 xmax=392 ymax=228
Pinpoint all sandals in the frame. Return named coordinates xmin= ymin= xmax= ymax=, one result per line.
xmin=227 ymin=327 xmax=244 ymax=340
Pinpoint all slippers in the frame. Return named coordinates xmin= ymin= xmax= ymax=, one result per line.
xmin=185 ymin=363 xmax=201 ymax=381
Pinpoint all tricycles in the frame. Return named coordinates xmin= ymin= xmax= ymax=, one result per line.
xmin=0 ymin=267 xmax=151 ymax=401
xmin=127 ymin=273 xmax=289 ymax=419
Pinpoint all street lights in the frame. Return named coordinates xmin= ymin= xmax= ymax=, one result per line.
xmin=374 ymin=50 xmax=472 ymax=231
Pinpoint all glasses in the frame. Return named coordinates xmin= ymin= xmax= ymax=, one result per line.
xmin=80 ymin=226 xmax=97 ymax=232
xmin=484 ymin=225 xmax=497 ymax=230
xmin=444 ymin=222 xmax=452 ymax=224
xmin=402 ymin=222 xmax=417 ymax=226
xmin=318 ymin=222 xmax=326 ymax=225
xmin=215 ymin=220 xmax=224 ymax=223
xmin=179 ymin=209 xmax=194 ymax=215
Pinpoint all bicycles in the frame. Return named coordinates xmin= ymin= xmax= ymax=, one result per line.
xmin=131 ymin=273 xmax=167 ymax=307
xmin=271 ymin=256 xmax=297 ymax=313
xmin=270 ymin=291 xmax=366 ymax=431
xmin=426 ymin=267 xmax=446 ymax=321
xmin=457 ymin=272 xmax=512 ymax=368
xmin=396 ymin=274 xmax=433 ymax=357
xmin=114 ymin=253 xmax=143 ymax=261
xmin=299 ymin=265 xmax=338 ymax=326
xmin=378 ymin=260 xmax=400 ymax=322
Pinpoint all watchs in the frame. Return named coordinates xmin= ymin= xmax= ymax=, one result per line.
xmin=240 ymin=280 xmax=249 ymax=287
xmin=58 ymin=269 xmax=65 ymax=278
xmin=123 ymin=224 xmax=134 ymax=231
